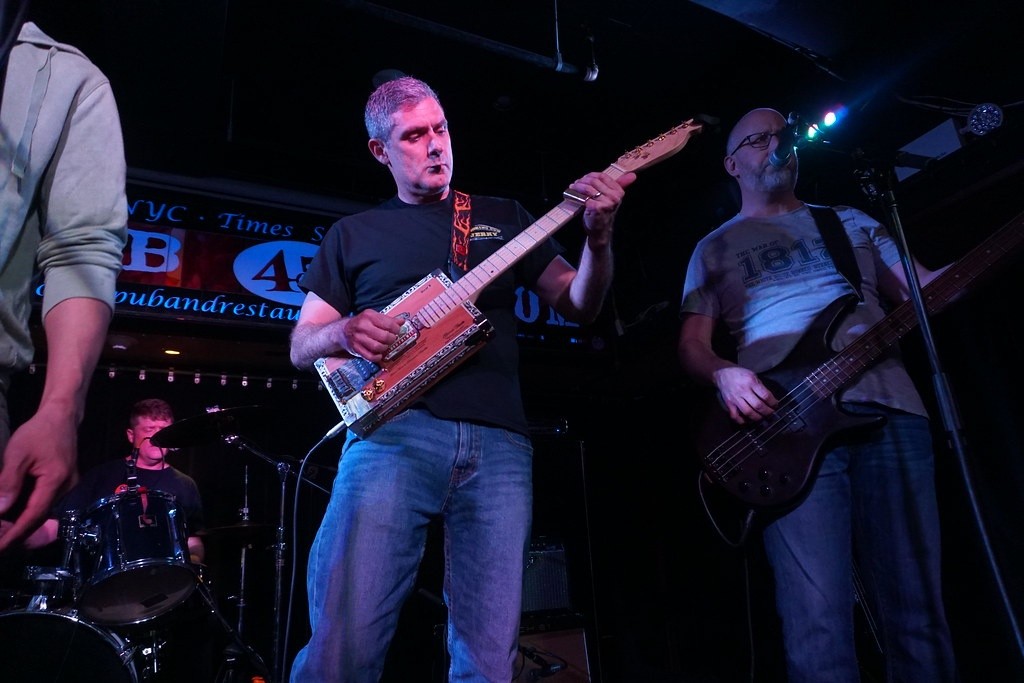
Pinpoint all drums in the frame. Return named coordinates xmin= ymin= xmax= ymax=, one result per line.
xmin=1 ymin=610 xmax=147 ymax=683
xmin=21 ymin=566 xmax=75 ymax=611
xmin=66 ymin=487 xmax=200 ymax=622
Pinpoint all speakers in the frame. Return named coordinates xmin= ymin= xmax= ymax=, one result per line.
xmin=520 ymin=540 xmax=571 ymax=624
xmin=492 ymin=623 xmax=592 ymax=683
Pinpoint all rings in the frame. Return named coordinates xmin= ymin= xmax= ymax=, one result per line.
xmin=591 ymin=192 xmax=602 ymax=200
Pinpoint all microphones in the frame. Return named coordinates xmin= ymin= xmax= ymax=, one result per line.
xmin=527 ymin=663 xmax=566 ymax=680
xmin=768 ymin=110 xmax=800 ymax=169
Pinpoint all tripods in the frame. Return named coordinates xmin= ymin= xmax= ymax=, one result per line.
xmin=217 ymin=430 xmax=329 ymax=683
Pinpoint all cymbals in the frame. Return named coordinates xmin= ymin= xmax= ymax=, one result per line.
xmin=152 ymin=406 xmax=277 ymax=444
xmin=208 ymin=523 xmax=276 ymax=533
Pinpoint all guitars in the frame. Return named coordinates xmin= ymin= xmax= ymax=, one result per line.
xmin=313 ymin=114 xmax=704 ymax=438
xmin=692 ymin=226 xmax=1022 ymax=512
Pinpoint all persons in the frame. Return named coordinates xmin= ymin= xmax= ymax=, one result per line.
xmin=678 ymin=107 xmax=970 ymax=683
xmin=288 ymin=76 xmax=637 ymax=683
xmin=61 ymin=399 xmax=207 ymax=573
xmin=0 ymin=13 xmax=129 ymax=556
